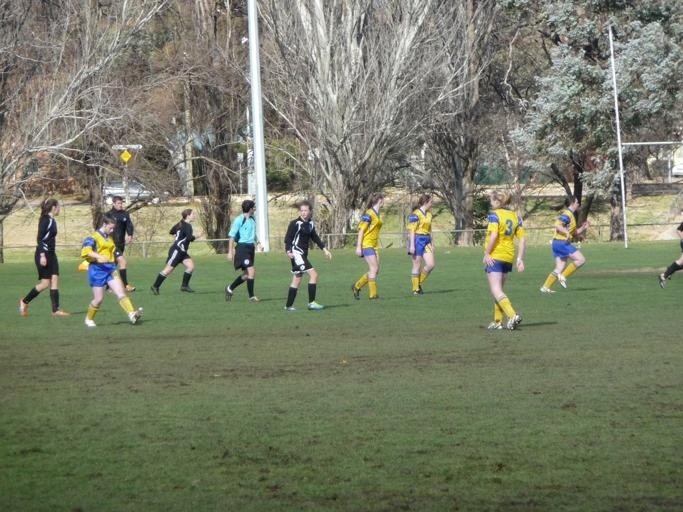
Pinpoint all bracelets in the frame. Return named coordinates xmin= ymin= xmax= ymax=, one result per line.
xmin=517 ymin=256 xmax=523 ymax=260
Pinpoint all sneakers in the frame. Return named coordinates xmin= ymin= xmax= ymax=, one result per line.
xmin=369 ymin=297 xmax=382 ymax=302
xmin=351 ymin=284 xmax=360 ymax=300
xmin=659 ymin=265 xmax=672 ymax=288
xmin=225 ymin=285 xmax=232 ymax=303
xmin=413 ymin=285 xmax=423 ymax=296
xmin=181 ymin=286 xmax=195 ymax=292
xmin=85 ymin=317 xmax=97 ymax=327
xmin=51 ymin=310 xmax=70 ymax=316
xmin=308 ymin=302 xmax=325 ymax=309
xmin=557 ymin=273 xmax=568 ymax=289
xmin=480 ymin=320 xmax=503 ymax=331
xmin=151 ymin=285 xmax=159 ymax=295
xmin=248 ymin=297 xmax=262 ymax=303
xmin=125 ymin=285 xmax=136 ymax=291
xmin=507 ymin=313 xmax=523 ymax=330
xmin=539 ymin=287 xmax=556 ymax=293
xmin=20 ymin=298 xmax=28 ymax=319
xmin=128 ymin=307 xmax=143 ymax=324
xmin=283 ymin=304 xmax=296 ymax=311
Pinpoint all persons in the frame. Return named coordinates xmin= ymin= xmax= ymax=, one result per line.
xmin=105 ymin=196 xmax=136 ymax=293
xmin=656 ymin=222 xmax=683 ymax=290
xmin=404 ymin=194 xmax=435 ymax=295
xmin=223 ymin=199 xmax=263 ymax=303
xmin=77 ymin=212 xmax=143 ymax=327
xmin=282 ymin=200 xmax=332 ymax=311
xmin=149 ymin=209 xmax=203 ymax=295
xmin=538 ymin=194 xmax=591 ymax=294
xmin=350 ymin=191 xmax=384 ymax=301
xmin=14 ymin=196 xmax=71 ymax=318
xmin=482 ymin=190 xmax=525 ymax=332
